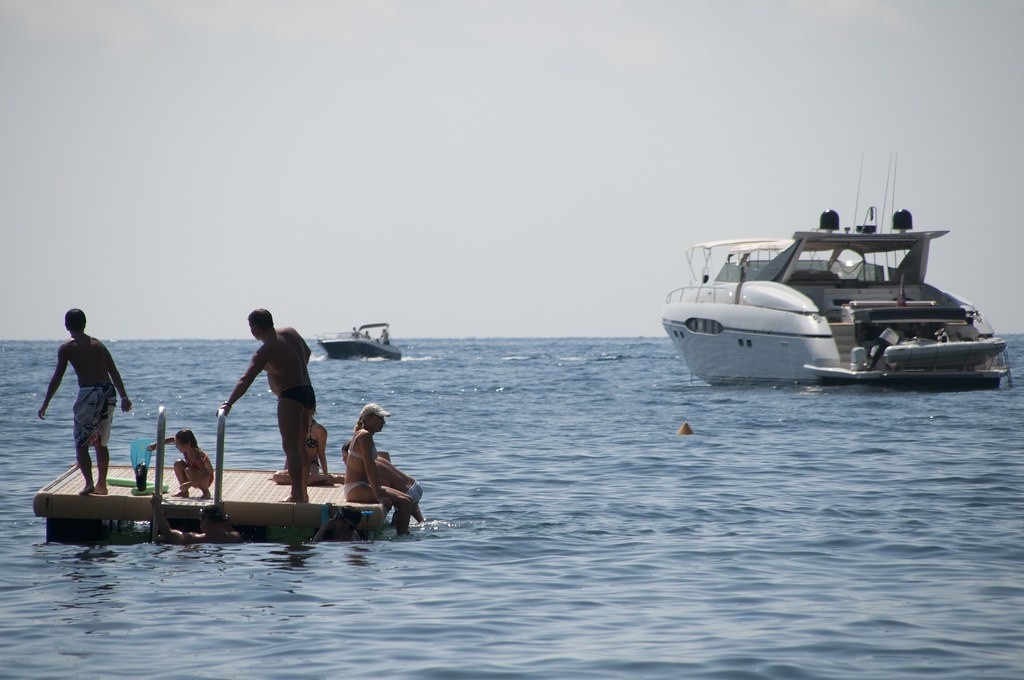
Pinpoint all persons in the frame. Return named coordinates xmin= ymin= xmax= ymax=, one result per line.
xmin=272 ymin=403 xmax=345 ymax=486
xmin=216 ymin=309 xmax=317 ymax=502
xmin=38 ymin=308 xmax=132 ymax=494
xmin=151 ymin=492 xmax=242 ymax=543
xmin=342 ymin=441 xmax=424 ymax=529
xmin=343 ymin=402 xmax=413 ymax=537
xmin=361 ymin=331 xmax=371 ymax=341
xmin=380 ymin=329 xmax=389 ymax=345
xmin=352 ymin=327 xmax=360 ymax=339
xmin=955 ymin=317 xmax=981 ymax=342
xmin=312 ymin=505 xmax=362 ymax=542
xmin=146 ymin=429 xmax=214 ymax=499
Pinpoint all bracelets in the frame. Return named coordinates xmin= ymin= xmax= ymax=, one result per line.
xmin=121 ymin=396 xmax=128 ymax=400
xmin=224 ymin=401 xmax=231 ymax=407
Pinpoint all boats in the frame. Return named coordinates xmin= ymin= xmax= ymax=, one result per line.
xmin=316 ymin=323 xmax=402 ymax=362
xmin=663 ymin=208 xmax=1013 ymax=391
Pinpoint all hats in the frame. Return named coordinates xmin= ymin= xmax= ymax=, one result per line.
xmin=361 ymin=403 xmax=391 ymax=420
